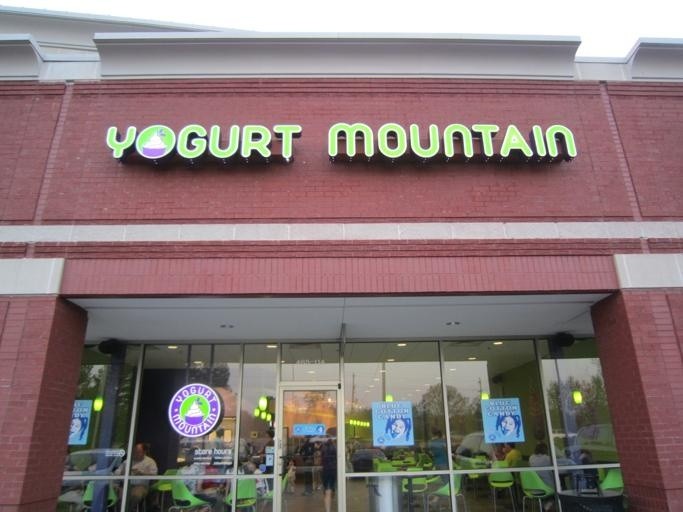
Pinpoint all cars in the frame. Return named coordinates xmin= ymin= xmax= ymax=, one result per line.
xmin=61 ymin=448 xmax=129 ymax=490
xmin=554 ymin=422 xmax=619 ymax=462
xmin=455 ymin=429 xmax=496 ymax=465
xmin=347 ymin=447 xmax=384 ymax=474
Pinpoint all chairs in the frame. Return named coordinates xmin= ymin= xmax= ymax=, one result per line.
xmin=55 ymin=468 xmax=289 ymax=512
xmin=364 ymin=448 xmax=623 ymax=512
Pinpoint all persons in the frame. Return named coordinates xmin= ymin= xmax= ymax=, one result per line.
xmin=68 ymin=416 xmax=87 ymax=440
xmin=495 ymin=416 xmax=520 ymax=438
xmin=57 ymin=423 xmax=599 ymax=512
xmin=384 ymin=416 xmax=411 ymax=440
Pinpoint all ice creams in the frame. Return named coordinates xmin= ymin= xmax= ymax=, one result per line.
xmin=143 ymin=136 xmax=166 ymax=157
xmin=185 ymin=403 xmax=204 ymax=425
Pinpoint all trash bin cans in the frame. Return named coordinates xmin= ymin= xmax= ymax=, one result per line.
xmin=368 ymin=477 xmax=403 ymax=512
